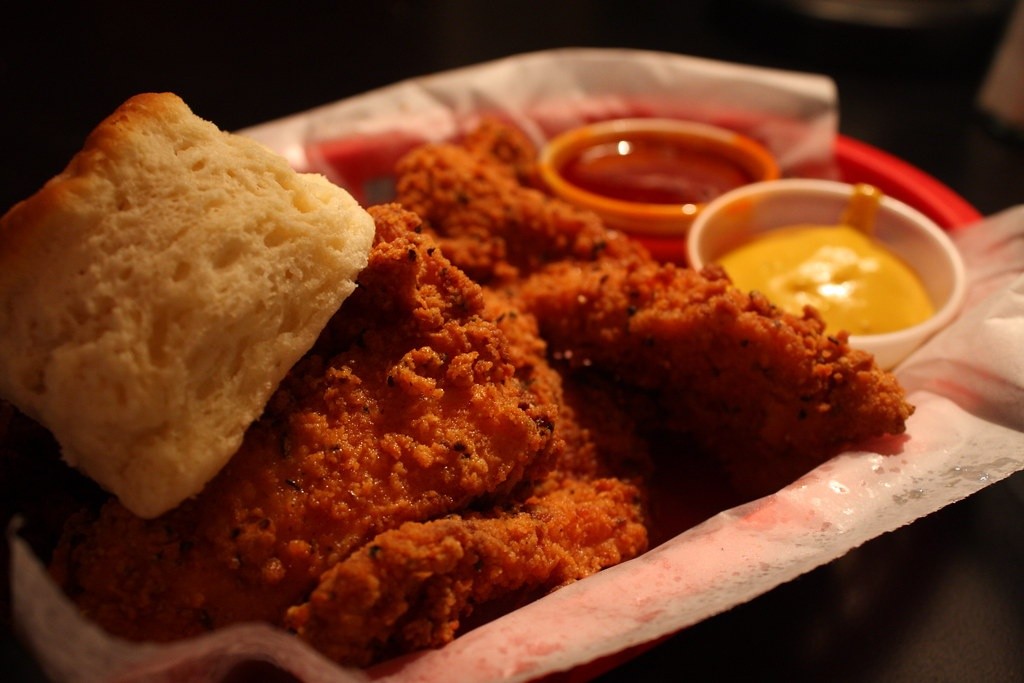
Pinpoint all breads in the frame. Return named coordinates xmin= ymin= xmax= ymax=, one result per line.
xmin=0 ymin=90 xmax=375 ymax=518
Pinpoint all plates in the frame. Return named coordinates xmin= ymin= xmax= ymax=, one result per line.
xmin=0 ymin=93 xmax=1024 ymax=683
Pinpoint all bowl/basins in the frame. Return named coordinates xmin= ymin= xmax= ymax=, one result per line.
xmin=537 ymin=118 xmax=781 ymax=266
xmin=687 ymin=178 xmax=970 ymax=373
